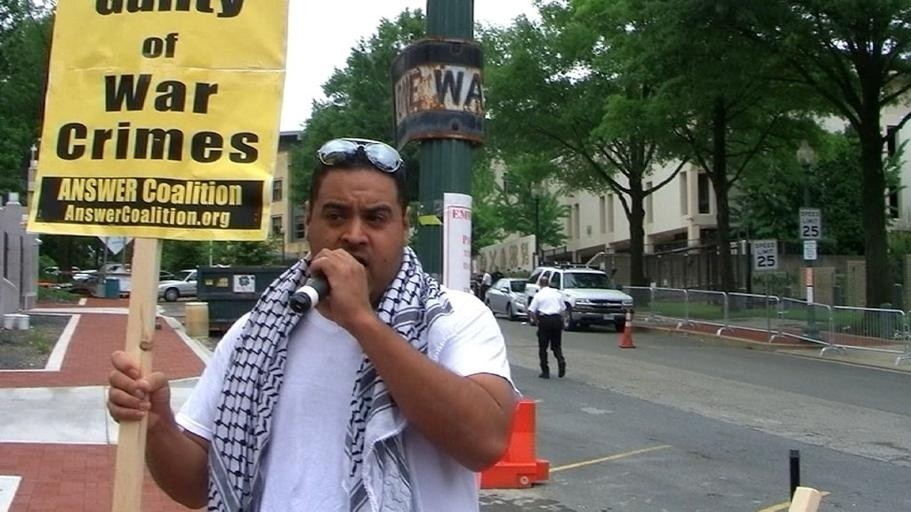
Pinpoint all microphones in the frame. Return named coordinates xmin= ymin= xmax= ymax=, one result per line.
xmin=289 ymin=274 xmax=329 ymax=317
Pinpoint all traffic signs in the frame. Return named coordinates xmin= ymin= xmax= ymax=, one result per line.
xmin=798 ymin=208 xmax=820 ymax=240
xmin=754 ymin=239 xmax=776 ymax=271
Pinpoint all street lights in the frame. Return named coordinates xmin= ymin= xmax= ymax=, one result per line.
xmin=527 ymin=178 xmax=544 ymax=267
xmin=798 ymin=137 xmax=822 ymax=345
xmin=279 ymin=227 xmax=286 ymax=264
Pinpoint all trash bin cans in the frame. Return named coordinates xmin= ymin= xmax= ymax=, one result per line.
xmin=184 ymin=302 xmax=210 ymax=339
xmin=106 ymin=279 xmax=120 ymax=299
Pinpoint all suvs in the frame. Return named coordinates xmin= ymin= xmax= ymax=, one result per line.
xmin=523 ymin=263 xmax=638 ymax=336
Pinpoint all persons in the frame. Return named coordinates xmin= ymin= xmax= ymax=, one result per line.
xmin=470 ymin=284 xmax=475 ymax=294
xmin=106 ymin=136 xmax=524 ymax=510
xmin=528 ymin=276 xmax=568 ymax=379
xmin=480 ymin=269 xmax=493 ymax=299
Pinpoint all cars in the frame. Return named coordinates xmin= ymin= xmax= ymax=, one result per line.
xmin=485 ymin=277 xmax=531 ymax=320
xmin=41 ymin=262 xmax=199 ymax=301
xmin=469 ymin=272 xmax=503 ymax=299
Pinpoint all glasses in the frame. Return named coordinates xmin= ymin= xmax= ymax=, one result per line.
xmin=317 ymin=137 xmax=404 ymax=173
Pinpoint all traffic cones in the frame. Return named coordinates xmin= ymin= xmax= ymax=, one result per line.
xmin=615 ymin=308 xmax=635 ymax=347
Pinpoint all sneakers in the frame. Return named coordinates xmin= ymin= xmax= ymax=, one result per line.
xmin=558 ymin=360 xmax=565 ymax=377
xmin=539 ymin=373 xmax=549 ymax=379
